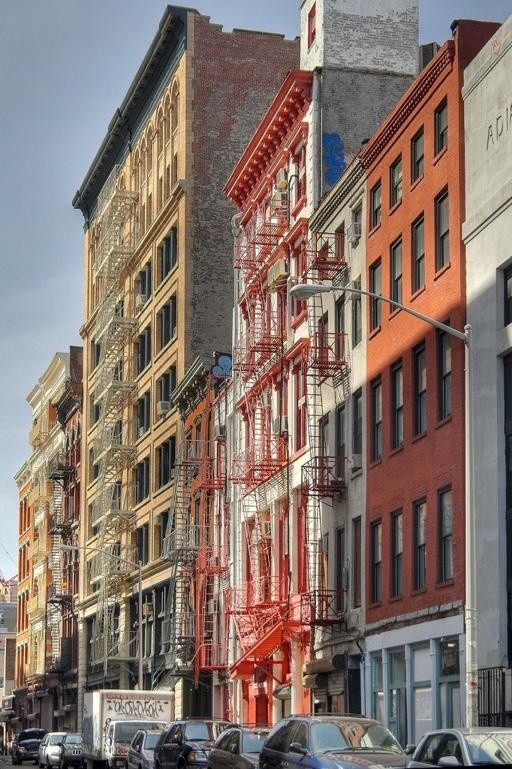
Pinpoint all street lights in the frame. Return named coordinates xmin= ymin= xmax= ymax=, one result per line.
xmin=60 ymin=545 xmax=143 ymax=690
xmin=288 ymin=284 xmax=478 ymax=728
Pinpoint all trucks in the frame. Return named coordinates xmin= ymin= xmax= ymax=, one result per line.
xmin=80 ymin=689 xmax=175 ymax=769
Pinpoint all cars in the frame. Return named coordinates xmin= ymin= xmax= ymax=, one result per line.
xmin=12 ymin=728 xmax=87 ymax=769
